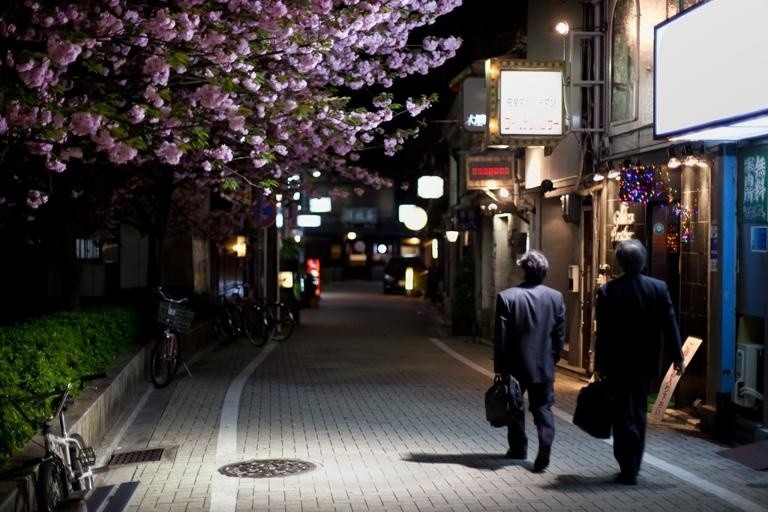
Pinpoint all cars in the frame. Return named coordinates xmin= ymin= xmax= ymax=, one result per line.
xmin=382 ymin=253 xmax=430 ymax=297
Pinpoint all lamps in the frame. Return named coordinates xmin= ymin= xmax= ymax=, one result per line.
xmin=665 ymin=142 xmax=698 ymax=169
xmin=591 ymin=161 xmax=621 ymax=183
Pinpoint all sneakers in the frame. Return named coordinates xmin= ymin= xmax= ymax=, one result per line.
xmin=613 ymin=471 xmax=640 ymax=485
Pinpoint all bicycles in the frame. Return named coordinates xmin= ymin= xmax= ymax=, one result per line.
xmin=0 ymin=370 xmax=109 ymax=512
xmin=146 ymin=265 xmax=300 ymax=390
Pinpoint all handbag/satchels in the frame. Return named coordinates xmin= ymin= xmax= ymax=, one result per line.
xmin=484 ymin=372 xmax=525 ymax=430
xmin=571 ymin=378 xmax=614 ymax=440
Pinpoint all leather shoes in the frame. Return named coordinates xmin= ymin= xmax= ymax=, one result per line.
xmin=533 ymin=444 xmax=552 ymax=473
xmin=507 ymin=448 xmax=527 ymax=459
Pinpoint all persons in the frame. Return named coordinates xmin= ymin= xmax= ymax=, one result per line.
xmin=489 ymin=250 xmax=565 ymax=473
xmin=301 ymin=272 xmax=316 ymax=309
xmin=590 ymin=237 xmax=686 ymax=487
